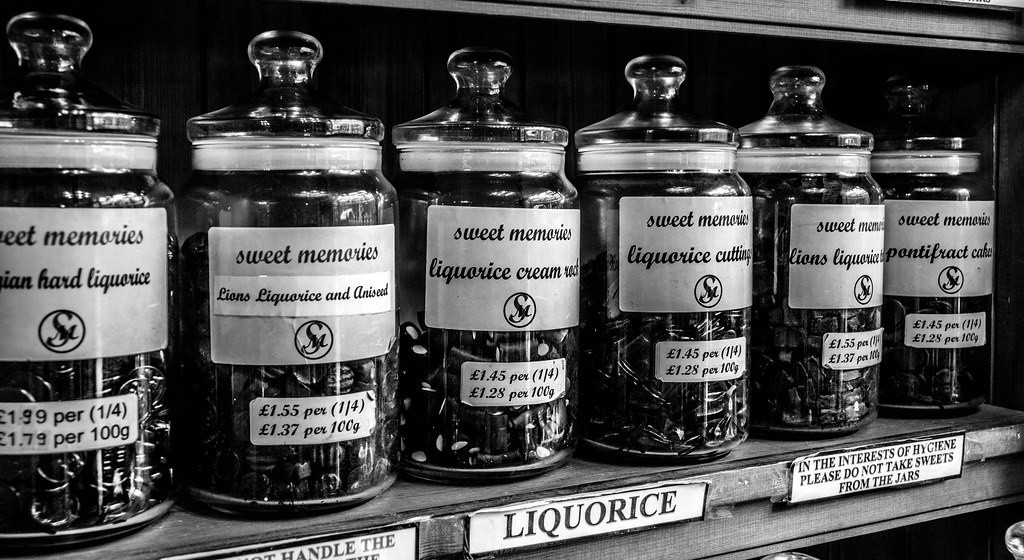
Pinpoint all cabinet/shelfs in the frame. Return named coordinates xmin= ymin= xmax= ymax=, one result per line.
xmin=0 ymin=0 xmax=1024 ymax=560
